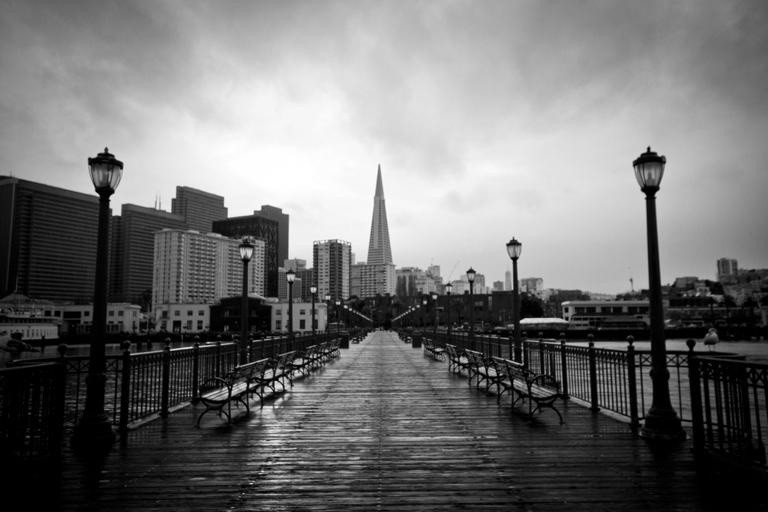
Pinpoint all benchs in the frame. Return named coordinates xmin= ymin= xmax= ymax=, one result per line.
xmin=192 ymin=335 xmax=366 ymax=433
xmin=399 ymin=331 xmax=563 ymax=427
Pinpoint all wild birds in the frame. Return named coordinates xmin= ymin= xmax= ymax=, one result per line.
xmin=704 ymin=327 xmax=719 ymax=350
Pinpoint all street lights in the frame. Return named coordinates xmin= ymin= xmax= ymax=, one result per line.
xmin=324 ymin=292 xmax=374 ymax=345
xmin=236 ymin=236 xmax=254 ymax=371
xmin=310 ymin=283 xmax=317 ymax=344
xmin=390 ymin=292 xmax=439 ymax=341
xmin=506 ymin=236 xmax=525 ymax=364
xmin=632 ymin=144 xmax=689 ymax=446
xmin=286 ymin=267 xmax=295 ymax=351
xmin=445 ymin=281 xmax=452 ymax=331
xmin=78 ymin=142 xmax=128 ymax=448
xmin=466 ymin=266 xmax=477 ymax=352
